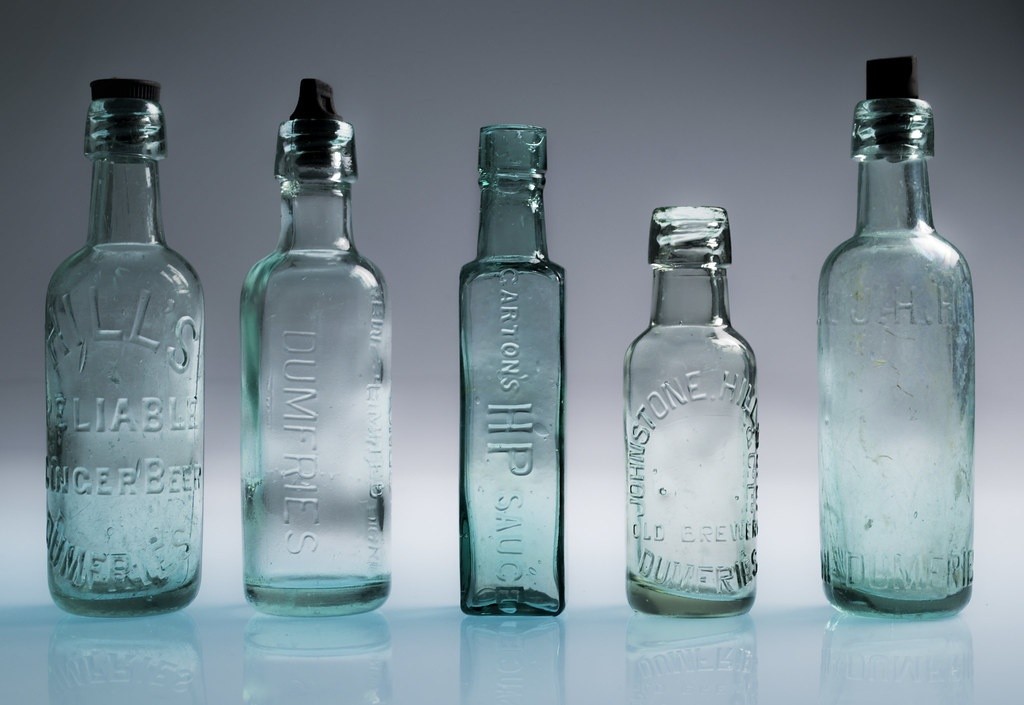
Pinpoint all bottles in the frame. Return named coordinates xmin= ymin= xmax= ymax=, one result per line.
xmin=47 ymin=76 xmax=203 ymax=614
xmin=238 ymin=78 xmax=391 ymax=614
xmin=818 ymin=615 xmax=976 ymax=705
xmin=458 ymin=125 xmax=565 ymax=619
xmin=815 ymin=55 xmax=975 ymax=619
xmin=240 ymin=615 xmax=392 ymax=705
xmin=460 ymin=618 xmax=566 ymax=705
xmin=624 ymin=205 xmax=755 ymax=618
xmin=627 ymin=610 xmax=757 ymax=705
xmin=51 ymin=616 xmax=204 ymax=705
